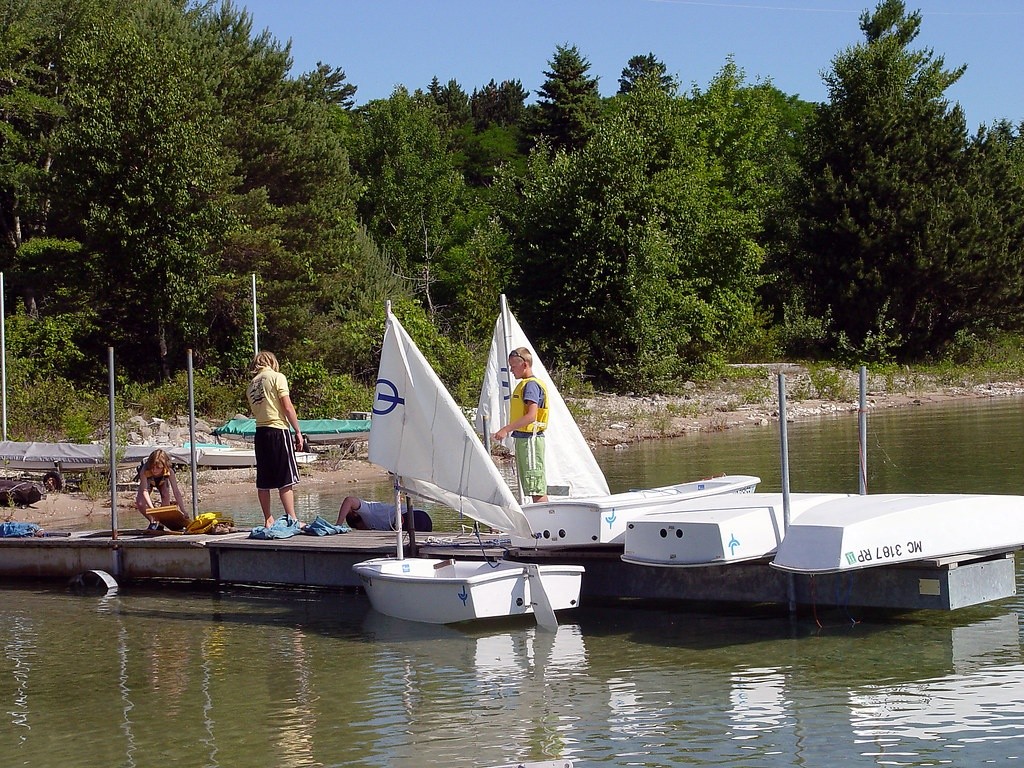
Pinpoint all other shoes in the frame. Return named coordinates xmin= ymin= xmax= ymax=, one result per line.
xmin=147 ymin=521 xmax=164 ymax=531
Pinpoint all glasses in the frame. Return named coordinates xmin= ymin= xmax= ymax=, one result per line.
xmin=509 ymin=350 xmax=526 ymax=362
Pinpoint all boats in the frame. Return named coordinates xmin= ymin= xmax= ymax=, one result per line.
xmin=507 ymin=476 xmax=761 ymax=546
xmin=353 ymin=554 xmax=584 ymax=635
xmin=769 ymin=492 xmax=1024 ymax=574
xmin=211 ymin=417 xmax=370 ymax=440
xmin=620 ymin=489 xmax=857 ymax=567
xmin=0 ymin=440 xmax=201 ymax=467
xmin=187 ymin=444 xmax=318 ymax=467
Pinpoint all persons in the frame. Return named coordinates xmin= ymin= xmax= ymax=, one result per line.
xmin=493 ymin=347 xmax=549 ymax=502
xmin=132 ymin=449 xmax=189 ymax=530
xmin=336 ymin=496 xmax=433 ymax=532
xmin=246 ymin=351 xmax=310 ymax=529
